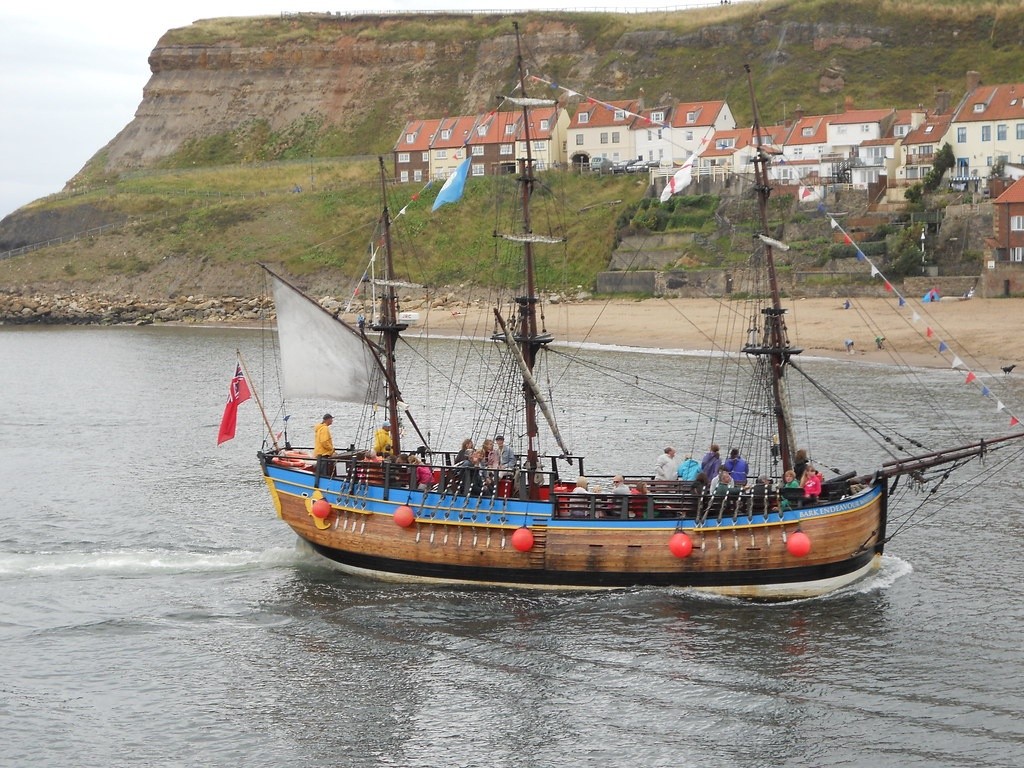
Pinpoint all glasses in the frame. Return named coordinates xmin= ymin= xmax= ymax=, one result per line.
xmin=613 ymin=481 xmax=622 ymax=483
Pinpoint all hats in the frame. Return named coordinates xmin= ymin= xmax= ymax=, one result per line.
xmin=383 ymin=421 xmax=391 ymax=426
xmin=496 ymin=436 xmax=504 ymax=439
xmin=758 ymin=475 xmax=772 ymax=481
xmin=323 ymin=414 xmax=335 ymax=419
xmin=576 ymin=476 xmax=589 ymax=492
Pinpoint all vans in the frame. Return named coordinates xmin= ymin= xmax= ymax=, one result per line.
xmin=591 ymin=157 xmax=608 ymax=171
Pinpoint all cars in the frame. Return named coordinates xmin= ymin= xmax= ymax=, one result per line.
xmin=627 ymin=161 xmax=660 ymax=173
xmin=609 ymin=160 xmax=633 ymax=173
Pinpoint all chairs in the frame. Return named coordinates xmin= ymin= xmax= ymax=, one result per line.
xmin=751 ymin=482 xmax=850 ymax=512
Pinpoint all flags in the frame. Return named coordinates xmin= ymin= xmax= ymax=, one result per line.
xmin=218 ymin=360 xmax=251 ymax=446
xmin=431 ymin=156 xmax=472 ymax=212
xmin=661 ymin=143 xmax=709 ymax=203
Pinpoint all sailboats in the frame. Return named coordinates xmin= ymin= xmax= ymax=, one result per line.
xmin=257 ymin=22 xmax=1024 ymax=598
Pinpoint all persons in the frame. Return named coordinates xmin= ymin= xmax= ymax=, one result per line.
xmin=358 ymin=314 xmax=363 ymax=322
xmin=875 ymin=335 xmax=886 ymax=350
xmin=653 ymin=444 xmax=824 ymax=518
xmin=845 ymin=338 xmax=855 ymax=352
xmin=570 ymin=474 xmax=671 ymax=518
xmin=453 ymin=434 xmax=546 ymax=496
xmin=926 ymin=286 xmax=938 ymax=302
xmin=356 ymin=420 xmax=434 ymax=490
xmin=968 ymin=286 xmax=975 ymax=299
xmin=313 ymin=414 xmax=338 ymax=476
xmin=843 ymin=300 xmax=850 ymax=309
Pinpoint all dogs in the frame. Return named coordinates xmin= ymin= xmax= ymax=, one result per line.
xmin=1000 ymin=365 xmax=1015 ymax=376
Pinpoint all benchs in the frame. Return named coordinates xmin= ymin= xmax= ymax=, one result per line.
xmin=623 ymin=479 xmax=747 ymax=494
xmin=554 ymin=495 xmax=737 ymax=518
xmin=327 ymin=462 xmax=420 ymax=490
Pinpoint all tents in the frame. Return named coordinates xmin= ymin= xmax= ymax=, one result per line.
xmin=922 ymin=291 xmax=940 ymax=303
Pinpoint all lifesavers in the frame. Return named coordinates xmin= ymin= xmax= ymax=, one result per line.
xmin=272 ymin=456 xmax=305 ymax=467
xmin=358 ymin=460 xmax=382 ymax=467
xmin=359 ymin=480 xmax=383 ymax=486
xmin=356 ymin=474 xmax=384 ymax=479
xmin=283 ymin=451 xmax=308 ymax=459
xmin=357 ymin=467 xmax=383 ymax=473
